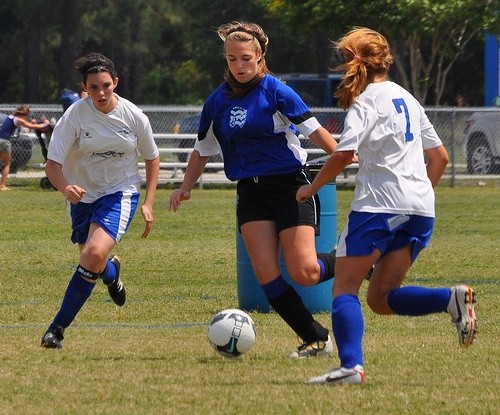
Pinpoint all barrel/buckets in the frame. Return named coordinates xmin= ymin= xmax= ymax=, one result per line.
xmin=236 ymin=165 xmax=337 ymax=313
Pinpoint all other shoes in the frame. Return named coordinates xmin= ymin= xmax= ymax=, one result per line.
xmin=0 ymin=184 xmax=10 ymax=191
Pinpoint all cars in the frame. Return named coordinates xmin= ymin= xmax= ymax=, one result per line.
xmin=0 ymin=111 xmax=32 ymax=168
xmin=464 ymin=95 xmax=500 ymax=175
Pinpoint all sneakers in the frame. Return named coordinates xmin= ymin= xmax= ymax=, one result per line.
xmin=289 ymin=333 xmax=334 ymax=360
xmin=334 ymin=234 xmax=375 ymax=281
xmin=40 ymin=327 xmax=63 ymax=351
xmin=450 ymin=284 xmax=478 ymax=350
xmin=106 ymin=255 xmax=127 ymax=308
xmin=308 ymin=362 xmax=364 ymax=387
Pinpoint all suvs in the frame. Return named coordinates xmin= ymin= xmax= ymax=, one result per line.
xmin=171 ymin=72 xmax=362 ymax=182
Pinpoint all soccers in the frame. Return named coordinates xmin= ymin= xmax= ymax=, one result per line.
xmin=207 ymin=309 xmax=255 ymax=357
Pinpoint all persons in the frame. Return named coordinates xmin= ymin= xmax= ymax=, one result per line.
xmin=0 ymin=85 xmax=200 ymax=194
xmin=40 ymin=54 xmax=160 ymax=347
xmin=168 ymin=21 xmax=375 ymax=359
xmin=296 ymin=26 xmax=478 ymax=384
xmin=0 ymin=106 xmax=48 ymax=191
